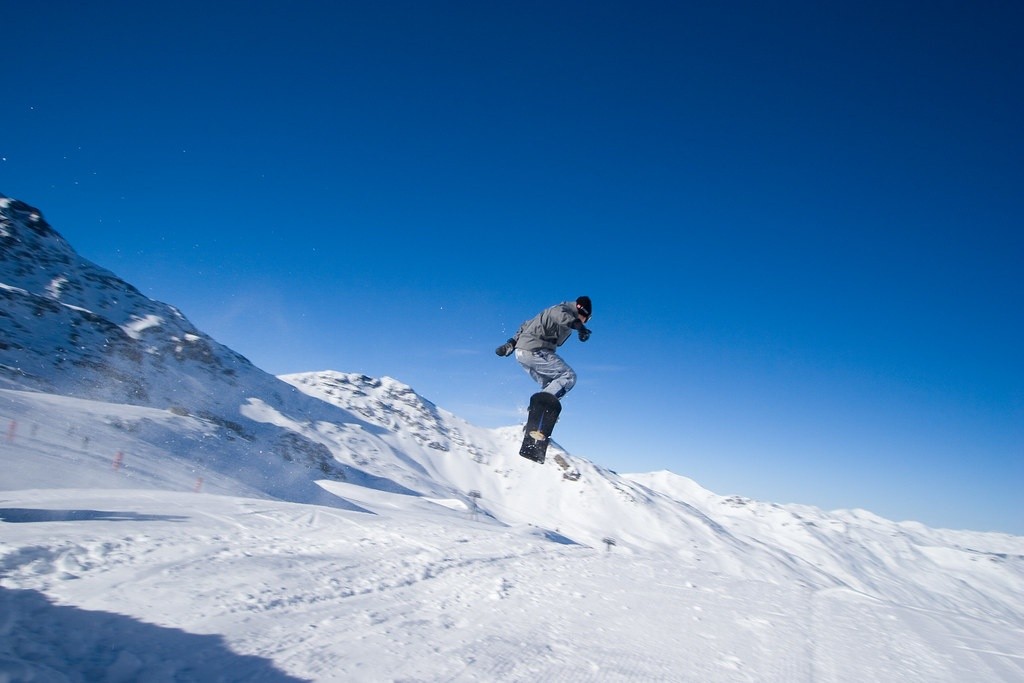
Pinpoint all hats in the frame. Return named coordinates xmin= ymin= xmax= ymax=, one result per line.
xmin=576 ymin=295 xmax=591 ymax=317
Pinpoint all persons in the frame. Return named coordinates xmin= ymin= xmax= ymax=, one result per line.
xmin=495 ymin=296 xmax=593 ymax=401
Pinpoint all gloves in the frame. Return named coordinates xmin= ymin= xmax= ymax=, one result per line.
xmin=496 ymin=339 xmax=516 ymax=356
xmin=579 ymin=326 xmax=590 ymax=342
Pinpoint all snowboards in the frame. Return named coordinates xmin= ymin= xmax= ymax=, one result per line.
xmin=519 ymin=392 xmax=563 ymax=465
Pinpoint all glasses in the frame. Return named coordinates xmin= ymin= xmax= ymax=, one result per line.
xmin=585 ymin=315 xmax=591 ymax=323
